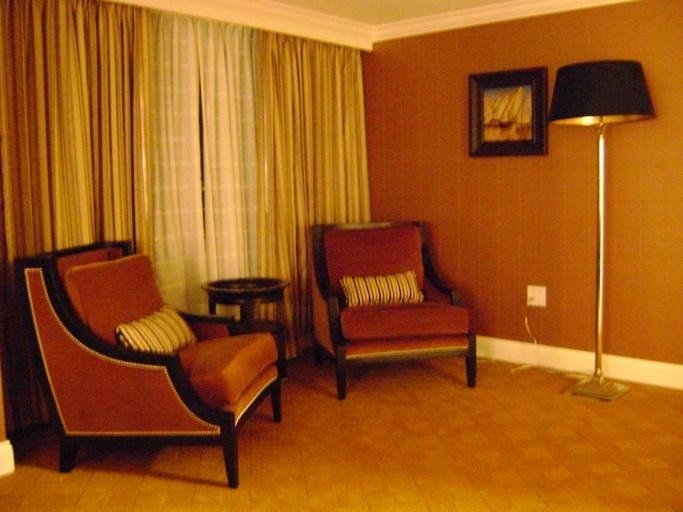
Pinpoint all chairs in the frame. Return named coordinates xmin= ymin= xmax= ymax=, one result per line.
xmin=306 ymin=218 xmax=482 ymax=403
xmin=10 ymin=240 xmax=284 ymax=488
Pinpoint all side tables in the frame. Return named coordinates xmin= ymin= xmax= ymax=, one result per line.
xmin=202 ymin=277 xmax=291 ymax=388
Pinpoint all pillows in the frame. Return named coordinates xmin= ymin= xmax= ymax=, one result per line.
xmin=112 ymin=304 xmax=198 ymax=367
xmin=339 ymin=269 xmax=424 ymax=307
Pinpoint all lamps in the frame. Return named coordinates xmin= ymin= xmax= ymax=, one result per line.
xmin=546 ymin=58 xmax=656 ymax=404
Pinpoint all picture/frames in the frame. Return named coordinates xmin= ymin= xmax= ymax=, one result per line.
xmin=468 ymin=65 xmax=549 ymax=158
